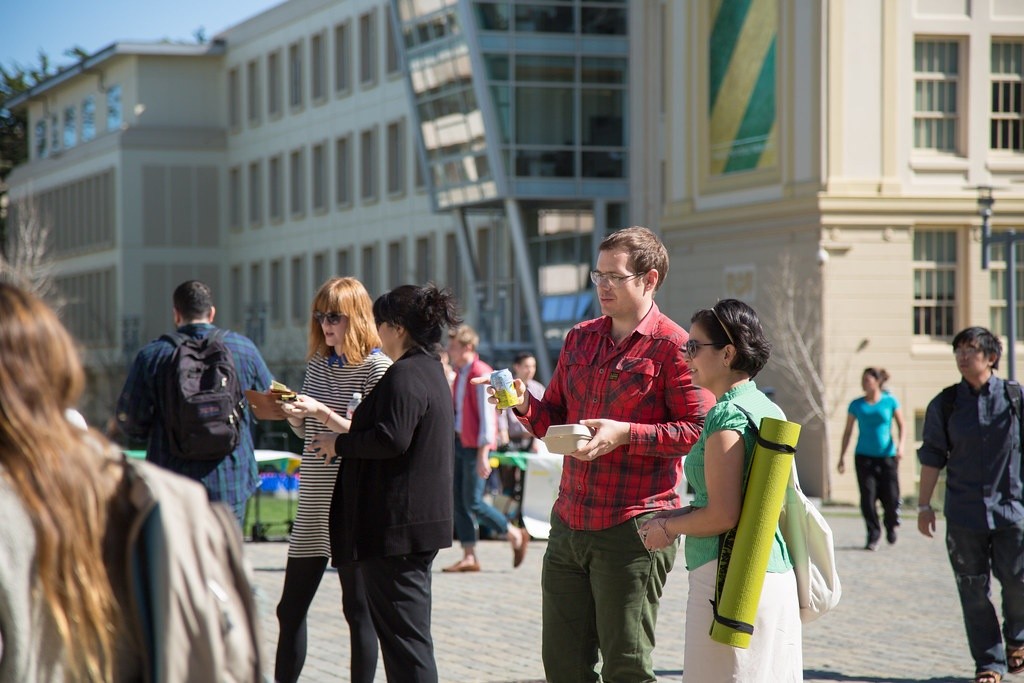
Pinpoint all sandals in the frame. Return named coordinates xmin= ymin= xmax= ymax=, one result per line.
xmin=1005 ymin=645 xmax=1024 ymax=673
xmin=975 ymin=671 xmax=1001 ymax=683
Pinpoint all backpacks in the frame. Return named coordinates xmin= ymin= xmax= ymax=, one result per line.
xmin=155 ymin=329 xmax=246 ymax=462
xmin=118 ymin=455 xmax=269 ymax=681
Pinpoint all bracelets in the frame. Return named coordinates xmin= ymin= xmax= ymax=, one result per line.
xmin=658 ymin=518 xmax=681 ymax=540
xmin=916 ymin=505 xmax=930 ymax=513
xmin=324 ymin=409 xmax=333 ymax=424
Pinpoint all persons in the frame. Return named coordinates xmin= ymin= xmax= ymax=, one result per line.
xmin=917 ymin=327 xmax=1024 ymax=683
xmin=306 ymin=281 xmax=465 ymax=683
xmin=0 ymin=262 xmax=263 ymax=683
xmin=436 ymin=324 xmax=549 ymax=571
xmin=470 ymin=226 xmax=717 ymax=683
xmin=838 ymin=367 xmax=905 ymax=550
xmin=115 ymin=281 xmax=275 ymax=532
xmin=638 ymin=298 xmax=804 ymax=683
xmin=275 ymin=277 xmax=396 ymax=683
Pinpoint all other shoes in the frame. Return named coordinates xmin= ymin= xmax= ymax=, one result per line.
xmin=887 ymin=527 xmax=896 ymax=544
xmin=513 ymin=528 xmax=529 ymax=567
xmin=442 ymin=561 xmax=480 ymax=572
xmin=865 ymin=540 xmax=876 ymax=551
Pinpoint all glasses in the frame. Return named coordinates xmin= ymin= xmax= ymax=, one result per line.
xmin=313 ymin=311 xmax=344 ymax=324
xmin=686 ymin=340 xmax=728 ymax=358
xmin=590 ymin=270 xmax=645 ymax=289
xmin=954 ymin=348 xmax=981 ymax=356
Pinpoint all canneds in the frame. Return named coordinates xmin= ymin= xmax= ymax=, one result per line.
xmin=490 ymin=368 xmax=519 ymax=409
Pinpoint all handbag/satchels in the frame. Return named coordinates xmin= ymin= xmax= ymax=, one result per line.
xmin=779 ymin=486 xmax=842 ymax=624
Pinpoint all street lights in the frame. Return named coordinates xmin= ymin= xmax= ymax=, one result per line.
xmin=965 ymin=156 xmax=1019 ymax=386
